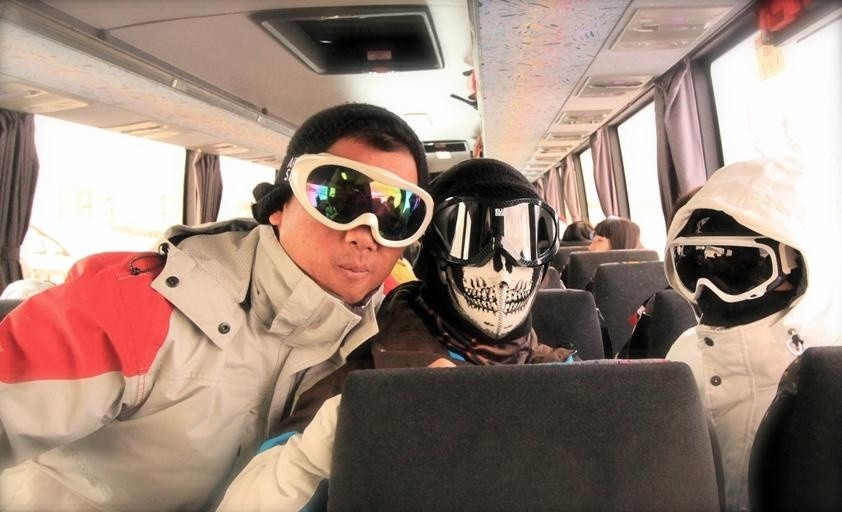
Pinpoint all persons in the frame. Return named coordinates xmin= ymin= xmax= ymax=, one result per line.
xmin=663 ymin=157 xmax=841 ymax=512
xmin=224 ymin=157 xmax=588 ymax=511
xmin=562 ymin=216 xmax=641 ymax=250
xmin=1 ymin=103 xmax=434 ymax=512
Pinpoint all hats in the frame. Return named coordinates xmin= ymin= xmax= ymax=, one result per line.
xmin=428 ymin=159 xmax=539 ymax=202
xmin=251 ymin=104 xmax=428 ymax=224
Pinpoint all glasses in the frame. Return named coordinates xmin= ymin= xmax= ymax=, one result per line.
xmin=433 ymin=196 xmax=560 ymax=266
xmin=669 ymin=236 xmax=798 ymax=303
xmin=288 ymin=153 xmax=435 ymax=247
